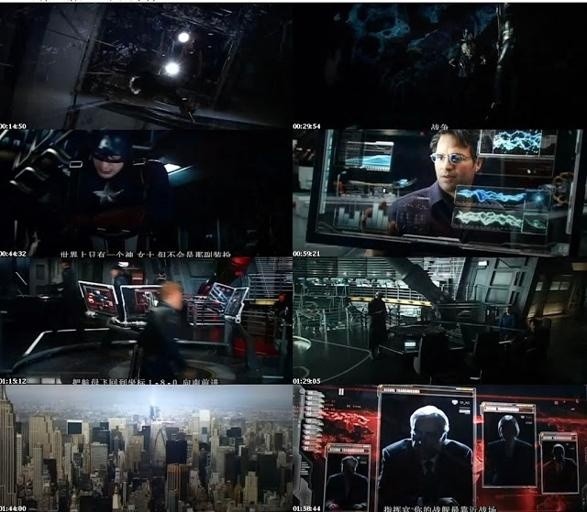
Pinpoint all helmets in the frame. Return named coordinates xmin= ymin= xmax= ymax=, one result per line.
xmin=91 ymin=131 xmax=131 ymax=153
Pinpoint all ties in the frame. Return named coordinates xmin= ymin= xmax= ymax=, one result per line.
xmin=423 ymin=461 xmax=433 ymax=475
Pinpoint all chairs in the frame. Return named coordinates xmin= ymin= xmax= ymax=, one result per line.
xmin=469 ymin=333 xmax=507 ymax=384
xmin=413 ymin=332 xmax=453 ymax=383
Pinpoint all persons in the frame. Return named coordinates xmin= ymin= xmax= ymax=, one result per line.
xmin=542 ymin=443 xmax=577 ymax=492
xmin=69 ymin=125 xmax=169 ymax=258
xmin=108 ymin=264 xmax=136 ymax=324
xmin=498 ymin=305 xmax=516 ymax=338
xmin=377 ymin=404 xmax=473 ymax=512
xmin=323 ymin=454 xmax=369 ymax=511
xmin=523 ymin=318 xmax=542 ymax=344
xmin=387 ymin=131 xmax=511 ymax=249
xmin=485 ymin=416 xmax=536 ymax=486
xmin=367 ymin=291 xmax=389 ymax=362
xmin=57 ymin=257 xmax=85 ymax=332
xmin=139 ymin=281 xmax=199 ymax=384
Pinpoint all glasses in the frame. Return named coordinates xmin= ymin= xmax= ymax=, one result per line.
xmin=429 ymin=153 xmax=472 ymax=164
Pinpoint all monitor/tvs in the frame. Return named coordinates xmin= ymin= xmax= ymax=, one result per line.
xmin=306 ymin=128 xmax=586 ymax=256
